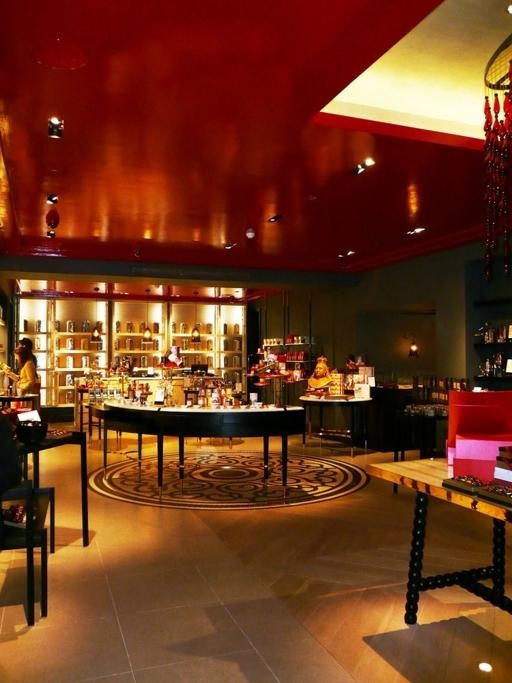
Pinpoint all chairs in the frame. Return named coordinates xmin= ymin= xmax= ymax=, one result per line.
xmin=0 ymin=414 xmax=55 ymax=626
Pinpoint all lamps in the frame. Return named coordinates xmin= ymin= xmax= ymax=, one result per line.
xmin=47 ymin=223 xmax=57 ymax=238
xmin=48 ymin=122 xmax=62 ymax=139
xmin=47 ymin=195 xmax=57 ymax=205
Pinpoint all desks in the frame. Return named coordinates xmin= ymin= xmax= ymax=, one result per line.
xmin=298 ymin=393 xmax=373 ymax=458
xmin=390 ymin=410 xmax=448 ymax=495
xmin=16 ymin=430 xmax=89 ymax=554
xmin=94 ymin=396 xmax=304 ymax=487
xmin=365 ymin=459 xmax=511 ymax=624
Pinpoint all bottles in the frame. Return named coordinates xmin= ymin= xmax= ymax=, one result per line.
xmin=123 ymin=377 xmax=264 ymax=410
xmin=405 ymin=375 xmax=466 ymax=418
xmin=88 ymin=388 xmax=122 ymax=406
xmin=262 ymin=336 xmax=309 ymax=382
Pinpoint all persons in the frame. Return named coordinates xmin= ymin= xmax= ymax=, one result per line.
xmin=6 ymin=347 xmax=35 ymax=393
xmin=8 ymin=338 xmax=37 ymax=380
xmin=308 ymin=356 xmax=333 ymax=388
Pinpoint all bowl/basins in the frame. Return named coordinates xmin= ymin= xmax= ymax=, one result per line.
xmin=16 ymin=421 xmax=48 ymax=445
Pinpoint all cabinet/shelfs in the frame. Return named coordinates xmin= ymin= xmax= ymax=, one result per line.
xmin=472 ymin=295 xmax=512 ymax=389
